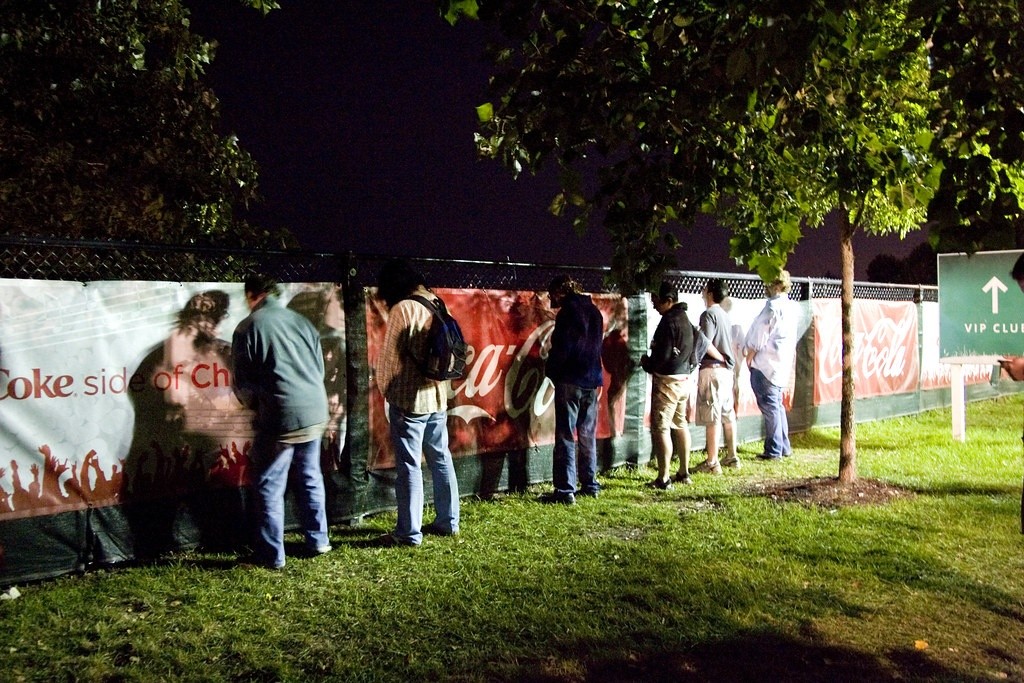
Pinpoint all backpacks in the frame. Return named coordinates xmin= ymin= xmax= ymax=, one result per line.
xmin=400 ymin=297 xmax=464 ymax=379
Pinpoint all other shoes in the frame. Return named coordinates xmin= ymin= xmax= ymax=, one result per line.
xmin=757 ymin=454 xmax=781 ymax=459
xmin=645 ymin=476 xmax=672 ymax=491
xmin=422 ymin=522 xmax=459 ymax=537
xmin=379 ymin=533 xmax=421 ymax=548
xmin=670 ymin=472 xmax=691 ymax=485
xmin=542 ymin=494 xmax=576 ymax=506
xmin=574 ymin=489 xmax=598 ymax=499
xmin=236 ymin=556 xmax=285 ymax=571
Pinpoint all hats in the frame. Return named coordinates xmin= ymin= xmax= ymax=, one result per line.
xmin=549 ymin=273 xmax=571 ymax=309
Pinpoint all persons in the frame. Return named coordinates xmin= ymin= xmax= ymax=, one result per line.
xmin=1000 ymin=252 xmax=1024 ymax=536
xmin=231 ymin=280 xmax=333 ymax=570
xmin=743 ymin=270 xmax=798 ymax=460
xmin=375 ymin=259 xmax=467 ymax=547
xmin=540 ymin=268 xmax=604 ymax=503
xmin=636 ymin=275 xmax=743 ymax=491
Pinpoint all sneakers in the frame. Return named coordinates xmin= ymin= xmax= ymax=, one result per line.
xmin=690 ymin=459 xmax=722 ymax=475
xmin=721 ymin=455 xmax=739 ymax=467
